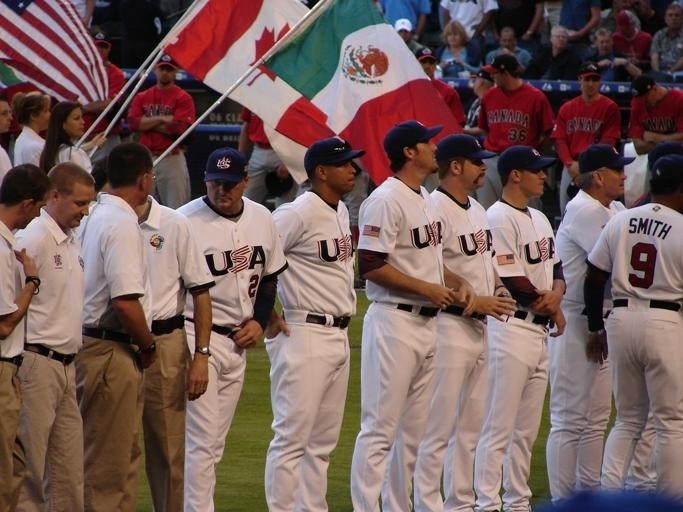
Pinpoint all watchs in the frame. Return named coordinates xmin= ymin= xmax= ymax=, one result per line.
xmin=194 ymin=346 xmax=213 ymax=356
xmin=495 ymin=283 xmax=507 ymax=291
xmin=138 ymin=341 xmax=156 ymax=356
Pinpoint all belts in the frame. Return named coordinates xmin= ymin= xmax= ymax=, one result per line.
xmin=397 ymin=303 xmax=440 ymax=317
xmin=151 ymin=315 xmax=185 ymax=335
xmin=307 ymin=314 xmax=351 ymax=329
xmin=514 ymin=310 xmax=550 ymax=325
xmin=612 ymin=299 xmax=681 ymax=312
xmin=0 ymin=354 xmax=24 ymax=366
xmin=580 ymin=305 xmax=610 ymax=322
xmin=444 ymin=303 xmax=486 ymax=320
xmin=25 ymin=344 xmax=76 ymax=365
xmin=186 ymin=317 xmax=240 ymax=338
xmin=83 ymin=326 xmax=130 ymax=342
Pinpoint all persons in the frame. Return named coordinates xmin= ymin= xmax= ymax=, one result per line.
xmin=14 ymin=162 xmax=96 ymax=512
xmin=354 ymin=118 xmax=474 ymax=511
xmin=377 ymin=1 xmax=683 ymax=84
xmin=464 ymin=52 xmax=556 ymax=214
xmin=553 ymin=61 xmax=621 ymax=215
xmin=235 ymin=101 xmax=297 ymax=211
xmin=93 ymin=158 xmax=213 ymax=511
xmin=413 ymin=133 xmax=518 ymax=511
xmin=584 ymin=155 xmax=682 ymax=498
xmin=72 ymin=28 xmax=126 ymax=170
xmin=38 ymin=100 xmax=95 ymax=178
xmin=71 ymin=142 xmax=159 ymax=511
xmin=0 ymin=164 xmax=51 ymax=512
xmin=414 ymin=47 xmax=468 ymax=192
xmin=474 ymin=144 xmax=567 ymax=511
xmin=126 ymin=54 xmax=196 ymax=210
xmin=171 ymin=148 xmax=290 ymax=510
xmin=65 ymin=0 xmax=198 ymax=65
xmin=546 ymin=141 xmax=631 ymax=503
xmin=0 ymin=94 xmax=15 ymax=185
xmin=466 ymin=66 xmax=494 ymax=152
xmin=627 ymin=74 xmax=683 ymax=205
xmin=264 ymin=136 xmax=365 ymax=511
xmin=12 ymin=91 xmax=52 ymax=168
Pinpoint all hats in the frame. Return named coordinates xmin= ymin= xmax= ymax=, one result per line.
xmin=394 ymin=18 xmax=413 ymax=34
xmin=647 ymin=141 xmax=683 ymax=167
xmin=496 ymin=145 xmax=557 ymax=174
xmin=415 ymin=46 xmax=438 ymax=61
xmin=204 ymin=146 xmax=248 ymax=182
xmin=155 ymin=52 xmax=178 ymax=70
xmin=435 ymin=133 xmax=496 ymax=161
xmin=579 ymin=144 xmax=636 ymax=171
xmin=614 ymin=9 xmax=637 ymax=34
xmin=631 ymin=76 xmax=656 ymax=94
xmin=383 ymin=120 xmax=443 ymax=157
xmin=303 ymin=136 xmax=366 ymax=173
xmin=481 ymin=53 xmax=520 ymax=74
xmin=650 ymin=156 xmax=683 ymax=189
xmin=91 ymin=30 xmax=113 ymax=46
xmin=577 ymin=62 xmax=602 ymax=78
